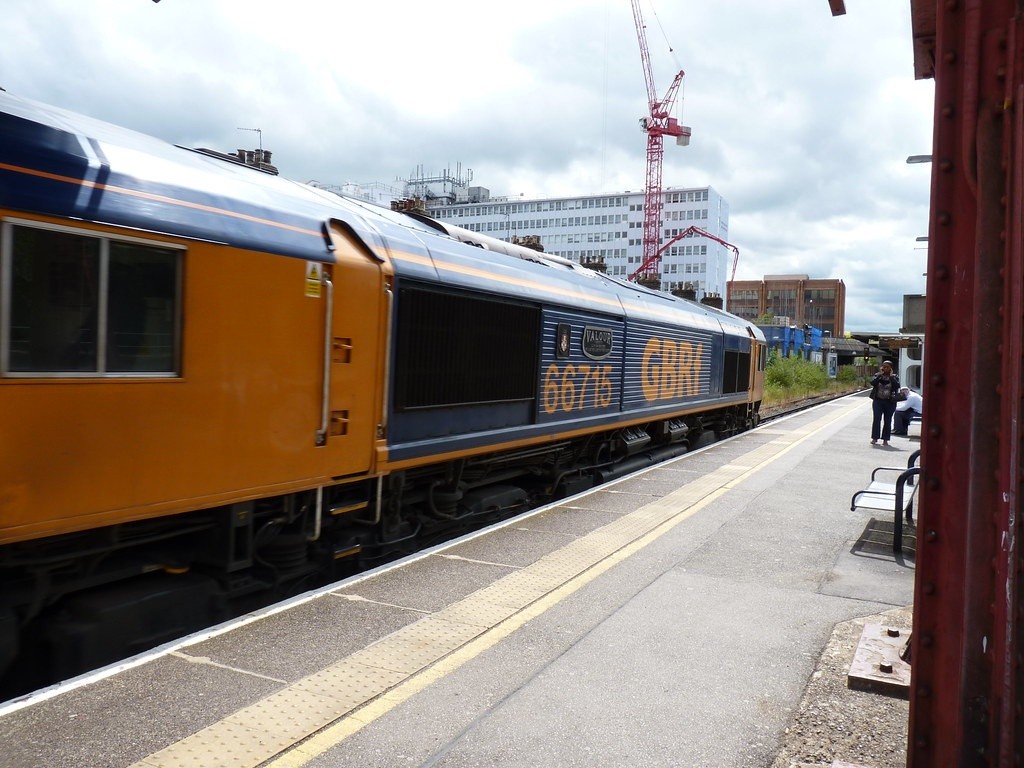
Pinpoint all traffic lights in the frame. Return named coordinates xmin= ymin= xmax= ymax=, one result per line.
xmin=864 ymin=348 xmax=869 ymax=362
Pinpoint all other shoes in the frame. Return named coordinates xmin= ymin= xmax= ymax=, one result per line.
xmin=871 ymin=439 xmax=877 ymax=444
xmin=891 ymin=429 xmax=908 ymax=436
xmin=882 ymin=442 xmax=888 ymax=445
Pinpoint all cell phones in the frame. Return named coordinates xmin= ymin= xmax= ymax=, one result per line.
xmin=882 ymin=369 xmax=884 ymax=371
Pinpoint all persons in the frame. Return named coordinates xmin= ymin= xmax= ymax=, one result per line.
xmin=869 ymin=361 xmax=900 ymax=446
xmin=891 ymin=388 xmax=923 ymax=435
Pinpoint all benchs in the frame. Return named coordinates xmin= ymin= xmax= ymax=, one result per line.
xmin=851 ymin=449 xmax=920 ymax=555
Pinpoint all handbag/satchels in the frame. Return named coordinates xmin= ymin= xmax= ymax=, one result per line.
xmin=891 ymin=391 xmax=906 ymax=401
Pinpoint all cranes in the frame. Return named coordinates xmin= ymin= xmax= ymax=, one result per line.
xmin=628 ymin=0 xmax=693 ymax=289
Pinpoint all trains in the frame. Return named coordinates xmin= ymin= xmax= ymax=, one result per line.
xmin=0 ymin=89 xmax=771 ymax=681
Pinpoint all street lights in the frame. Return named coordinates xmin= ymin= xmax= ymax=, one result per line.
xmin=825 ymin=330 xmax=832 ymax=354
